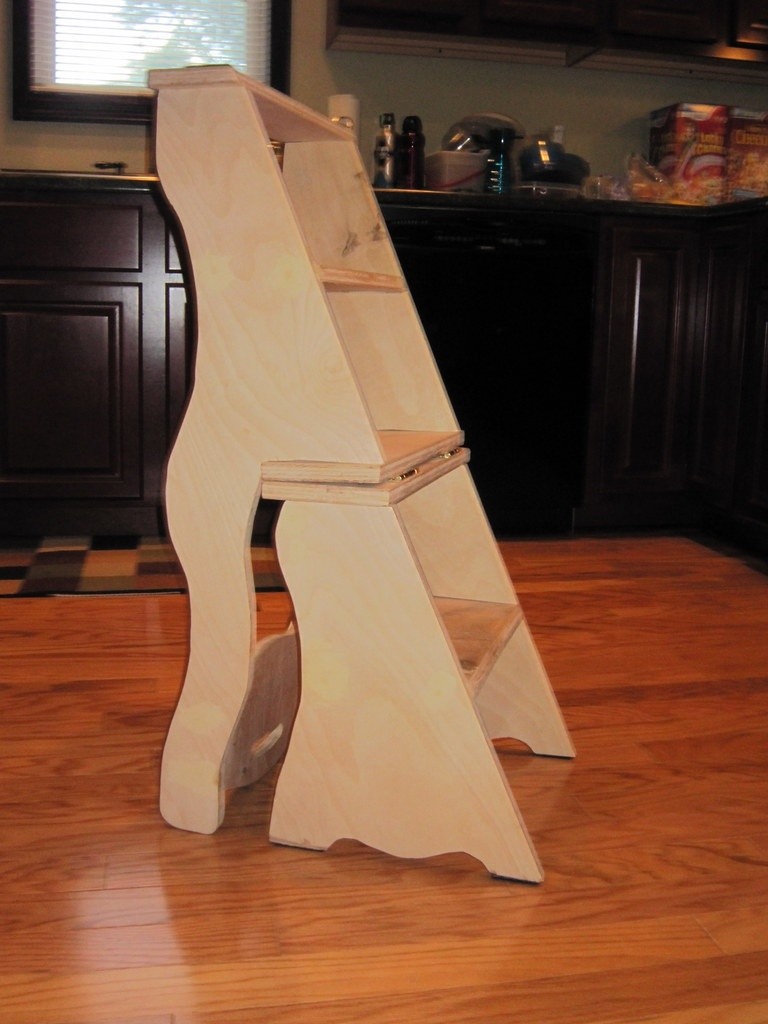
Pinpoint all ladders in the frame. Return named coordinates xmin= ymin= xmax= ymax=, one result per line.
xmin=147 ymin=65 xmax=578 ymax=884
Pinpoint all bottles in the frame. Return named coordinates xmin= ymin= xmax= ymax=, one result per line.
xmin=399 ymin=115 xmax=426 ymax=189
xmin=374 ymin=113 xmax=400 ymax=188
xmin=272 ymin=141 xmax=283 ymax=171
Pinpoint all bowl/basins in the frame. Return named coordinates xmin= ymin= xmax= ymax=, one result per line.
xmin=508 ymin=181 xmax=580 ymax=201
xmin=582 ymin=175 xmax=614 ymax=200
xmin=424 ymin=150 xmax=490 ymax=191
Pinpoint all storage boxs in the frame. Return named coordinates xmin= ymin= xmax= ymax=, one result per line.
xmin=648 ymin=102 xmax=768 ymax=205
xmin=424 ymin=151 xmax=488 ymax=191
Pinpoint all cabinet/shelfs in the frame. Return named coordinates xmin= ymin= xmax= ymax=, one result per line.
xmin=324 ymin=0 xmax=768 ymax=87
xmin=0 ymin=190 xmax=767 ymax=572
